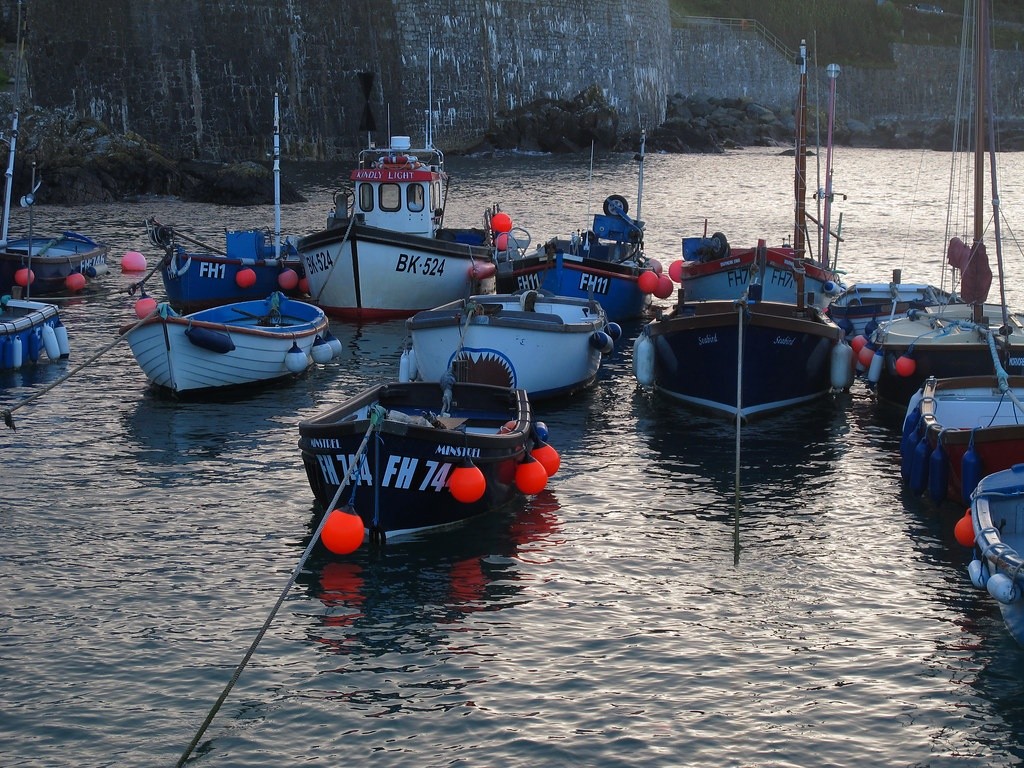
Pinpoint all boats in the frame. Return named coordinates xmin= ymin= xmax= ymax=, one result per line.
xmin=826 ymin=0 xmax=1024 ymax=653
xmin=631 ymin=38 xmax=855 ymax=422
xmin=0 ymin=161 xmax=70 ymax=374
xmin=399 ymin=288 xmax=623 ymax=402
xmin=119 ymin=291 xmax=343 ymax=397
xmin=1 ymin=111 xmax=111 ymax=296
xmin=145 ymin=30 xmax=848 ymax=323
xmin=299 ymin=360 xmax=560 ymax=555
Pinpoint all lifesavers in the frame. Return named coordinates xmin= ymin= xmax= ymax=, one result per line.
xmin=376 ymin=156 xmax=421 ymax=169
